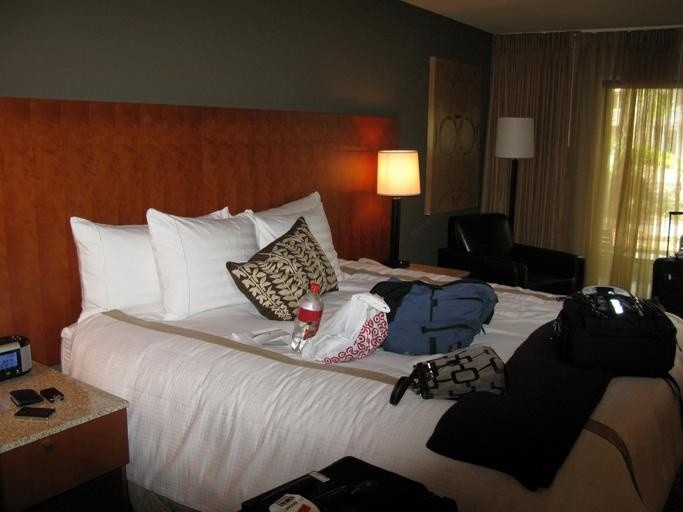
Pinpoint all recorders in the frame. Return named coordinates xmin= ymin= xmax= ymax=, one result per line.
xmin=0 ymin=334 xmax=32 ymax=381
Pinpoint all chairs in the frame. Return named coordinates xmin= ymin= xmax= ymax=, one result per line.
xmin=439 ymin=209 xmax=587 ymax=297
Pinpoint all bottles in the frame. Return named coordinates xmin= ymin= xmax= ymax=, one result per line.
xmin=288 ymin=283 xmax=324 ymax=352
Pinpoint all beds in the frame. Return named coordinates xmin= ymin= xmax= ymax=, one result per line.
xmin=57 ymin=187 xmax=682 ymax=512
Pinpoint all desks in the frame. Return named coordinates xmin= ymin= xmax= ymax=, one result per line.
xmin=647 ymin=254 xmax=682 ymax=315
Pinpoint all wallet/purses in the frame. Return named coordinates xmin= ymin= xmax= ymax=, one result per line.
xmin=8 ymin=388 xmax=46 ymax=408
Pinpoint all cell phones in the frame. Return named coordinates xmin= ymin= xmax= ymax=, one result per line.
xmin=15 ymin=406 xmax=56 ymax=421
xmin=40 ymin=387 xmax=64 ymax=402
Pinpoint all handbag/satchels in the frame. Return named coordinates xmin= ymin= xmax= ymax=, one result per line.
xmin=389 ymin=344 xmax=507 ymax=406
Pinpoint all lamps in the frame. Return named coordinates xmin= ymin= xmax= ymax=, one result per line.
xmin=375 ymin=147 xmax=423 ymax=270
xmin=494 ymin=116 xmax=536 ymax=241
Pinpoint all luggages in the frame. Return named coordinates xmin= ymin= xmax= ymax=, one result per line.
xmin=650 ymin=211 xmax=683 ymax=319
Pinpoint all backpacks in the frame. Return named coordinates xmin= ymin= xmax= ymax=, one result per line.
xmin=552 ymin=284 xmax=678 ymax=375
xmin=366 ymin=275 xmax=500 ymax=356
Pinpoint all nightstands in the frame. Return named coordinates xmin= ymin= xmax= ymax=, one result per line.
xmin=0 ymin=356 xmax=134 ymax=511
xmin=402 ymin=262 xmax=473 ymax=287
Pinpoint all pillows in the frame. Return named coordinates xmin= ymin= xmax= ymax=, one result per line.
xmin=243 ymin=191 xmax=344 ymax=284
xmin=224 ymin=215 xmax=340 ymax=321
xmin=63 ymin=198 xmax=231 ymax=326
xmin=142 ymin=204 xmax=261 ymax=326
